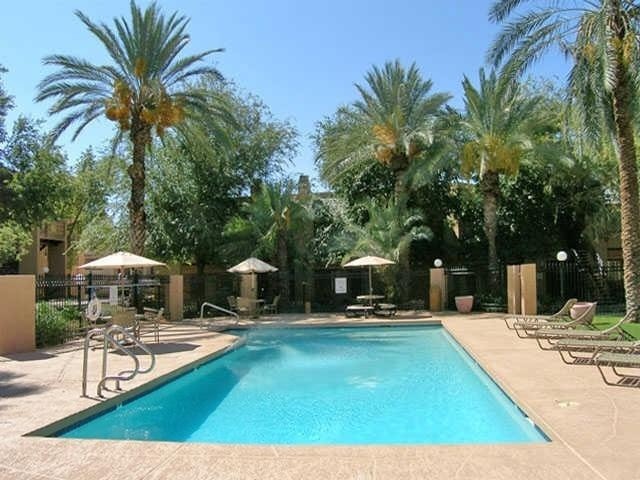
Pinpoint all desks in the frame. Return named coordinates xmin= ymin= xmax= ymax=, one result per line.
xmin=356 ymin=295 xmax=385 ymax=319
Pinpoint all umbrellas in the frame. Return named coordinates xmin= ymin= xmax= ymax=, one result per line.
xmin=77 ymin=249 xmax=167 ymax=340
xmin=344 ymin=254 xmax=397 ymax=314
xmin=226 ymin=254 xmax=280 ymax=315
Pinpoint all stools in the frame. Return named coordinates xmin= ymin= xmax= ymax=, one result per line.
xmin=345 ymin=303 xmax=398 ymax=318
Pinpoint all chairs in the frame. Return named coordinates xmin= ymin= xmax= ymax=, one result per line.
xmin=502 ymin=297 xmax=640 ymax=388
xmin=84 ymin=308 xmax=165 ymax=354
xmin=226 ymin=295 xmax=281 ymax=319
